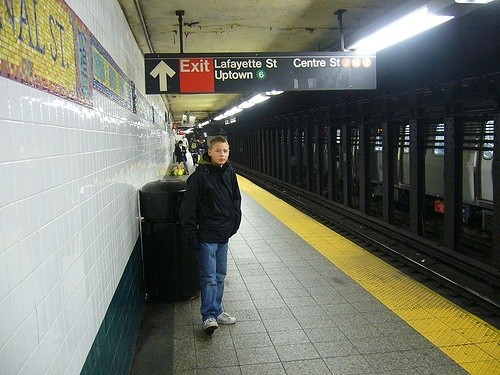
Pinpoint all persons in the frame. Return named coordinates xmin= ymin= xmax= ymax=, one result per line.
xmin=180 ymin=135 xmax=242 ymax=332
xmin=175 ymin=134 xmax=207 ymax=175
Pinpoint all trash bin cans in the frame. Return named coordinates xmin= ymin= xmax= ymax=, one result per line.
xmin=139 ymin=177 xmax=203 ymax=304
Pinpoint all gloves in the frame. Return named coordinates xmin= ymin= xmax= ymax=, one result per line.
xmin=189 ymin=235 xmax=201 ymax=249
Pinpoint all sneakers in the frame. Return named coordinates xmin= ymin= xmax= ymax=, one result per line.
xmin=203 ymin=318 xmax=218 ymax=331
xmin=217 ymin=312 xmax=237 ymax=324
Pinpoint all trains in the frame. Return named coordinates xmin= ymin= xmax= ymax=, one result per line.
xmin=303 ymin=121 xmax=495 ymax=228
